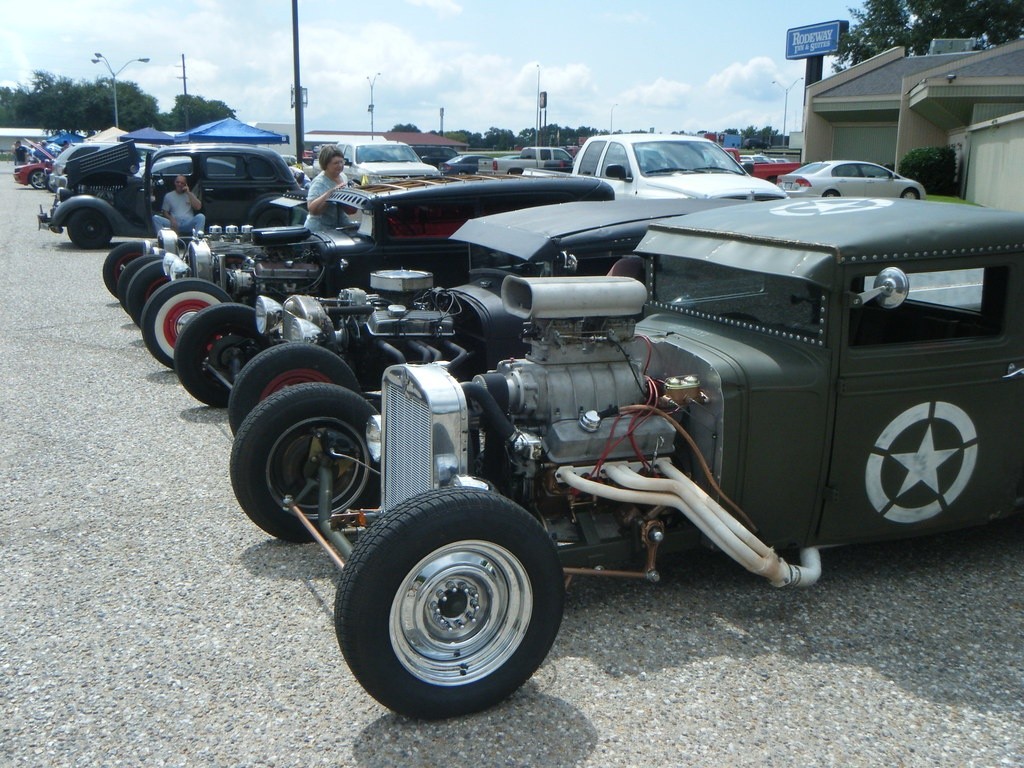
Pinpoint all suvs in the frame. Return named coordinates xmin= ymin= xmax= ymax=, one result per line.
xmin=35 ymin=143 xmax=313 ymax=250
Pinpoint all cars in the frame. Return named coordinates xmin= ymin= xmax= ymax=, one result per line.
xmin=14 ymin=139 xmax=790 ymax=446
xmin=438 ymin=154 xmax=493 ymax=178
xmin=228 ymin=199 xmax=1024 ymax=719
xmin=719 ymin=147 xmax=802 ymax=186
xmin=776 ymin=157 xmax=928 ymax=202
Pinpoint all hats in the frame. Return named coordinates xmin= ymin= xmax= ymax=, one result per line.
xmin=61 ymin=141 xmax=69 ymax=145
xmin=41 ymin=140 xmax=49 ymax=146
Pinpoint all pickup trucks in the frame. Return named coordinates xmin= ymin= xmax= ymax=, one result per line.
xmin=477 ymin=144 xmax=575 ymax=177
xmin=522 ymin=133 xmax=792 ymax=204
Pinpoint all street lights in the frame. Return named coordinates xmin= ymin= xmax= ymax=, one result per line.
xmin=366 ymin=72 xmax=381 ymax=141
xmin=772 ymin=76 xmax=805 ymax=149
xmin=90 ymin=52 xmax=152 ymax=127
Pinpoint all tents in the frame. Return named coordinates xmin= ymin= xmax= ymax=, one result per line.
xmin=46 ymin=117 xmax=289 ymax=146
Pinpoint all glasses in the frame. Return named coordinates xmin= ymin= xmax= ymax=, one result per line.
xmin=328 ymin=159 xmax=346 ymax=167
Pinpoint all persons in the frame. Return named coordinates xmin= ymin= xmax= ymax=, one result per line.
xmin=11 ymin=139 xmax=69 ymax=169
xmin=285 ymin=159 xmax=311 ymax=190
xmin=153 ymin=176 xmax=206 ymax=239
xmin=304 ymin=145 xmax=358 ymax=233
